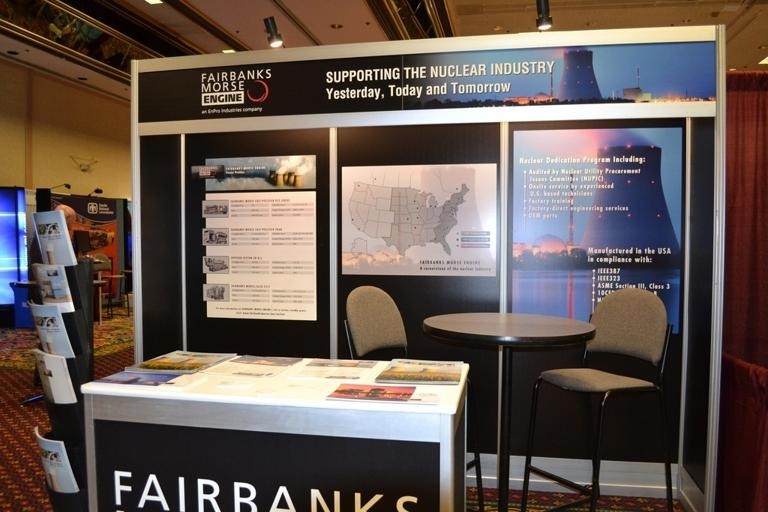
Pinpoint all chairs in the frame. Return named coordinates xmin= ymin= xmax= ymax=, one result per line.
xmin=100 ymin=256 xmax=114 ymax=318
xmin=342 ymin=282 xmax=485 ymax=512
xmin=520 ymin=281 xmax=673 ymax=512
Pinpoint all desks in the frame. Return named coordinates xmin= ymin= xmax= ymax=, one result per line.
xmin=422 ymin=311 xmax=595 ymax=512
xmin=78 ymin=348 xmax=470 ymax=512
xmin=92 ymin=277 xmax=115 ymax=321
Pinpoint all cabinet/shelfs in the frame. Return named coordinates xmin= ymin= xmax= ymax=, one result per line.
xmin=31 ymin=256 xmax=106 ymax=511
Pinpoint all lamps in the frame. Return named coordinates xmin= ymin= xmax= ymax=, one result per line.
xmin=87 ymin=187 xmax=103 ymax=197
xmin=531 ymin=0 xmax=554 ymax=35
xmin=50 ymin=183 xmax=71 ymax=190
xmin=261 ymin=16 xmax=285 ymax=51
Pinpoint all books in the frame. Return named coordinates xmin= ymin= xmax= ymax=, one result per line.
xmin=375 ymin=356 xmax=466 ymax=387
xmin=30 ymin=263 xmax=77 ymax=315
xmin=30 ymin=348 xmax=80 ymax=406
xmin=28 ymin=303 xmax=77 ymax=360
xmin=123 ymin=348 xmax=241 ymax=377
xmin=32 ymin=210 xmax=80 ymax=267
xmin=33 ymin=425 xmax=81 ymax=494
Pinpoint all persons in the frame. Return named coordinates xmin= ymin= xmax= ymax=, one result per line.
xmin=30 ymin=203 xmax=83 ymax=389
